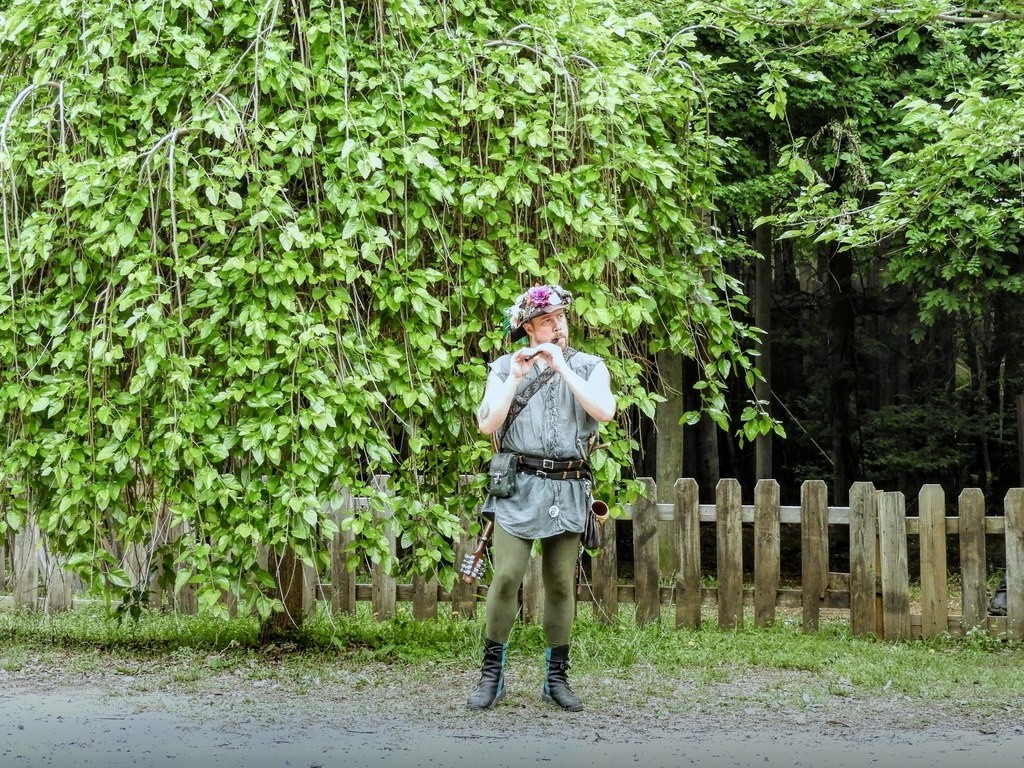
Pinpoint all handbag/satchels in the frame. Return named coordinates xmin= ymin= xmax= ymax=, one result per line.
xmin=489 ymin=452 xmax=517 ymax=496
xmin=581 ymin=485 xmax=601 ymax=551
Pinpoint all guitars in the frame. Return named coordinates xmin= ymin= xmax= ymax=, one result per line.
xmin=460 ymin=430 xmax=502 ymax=584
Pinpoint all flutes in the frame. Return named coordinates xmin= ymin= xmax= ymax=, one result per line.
xmin=524 ymin=337 xmax=559 ymax=362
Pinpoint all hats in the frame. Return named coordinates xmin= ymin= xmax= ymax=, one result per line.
xmin=510 ymin=286 xmax=573 ymax=343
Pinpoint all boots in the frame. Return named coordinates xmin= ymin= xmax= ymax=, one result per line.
xmin=467 ymin=638 xmax=506 ymax=711
xmin=543 ymin=645 xmax=583 ymax=713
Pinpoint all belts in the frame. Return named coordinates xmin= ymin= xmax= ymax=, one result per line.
xmin=516 ymin=454 xmax=593 ymax=481
xmin=518 ymin=466 xmax=591 ymax=481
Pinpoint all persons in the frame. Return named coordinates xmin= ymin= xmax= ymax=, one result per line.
xmin=462 ymin=283 xmax=616 ymax=714
